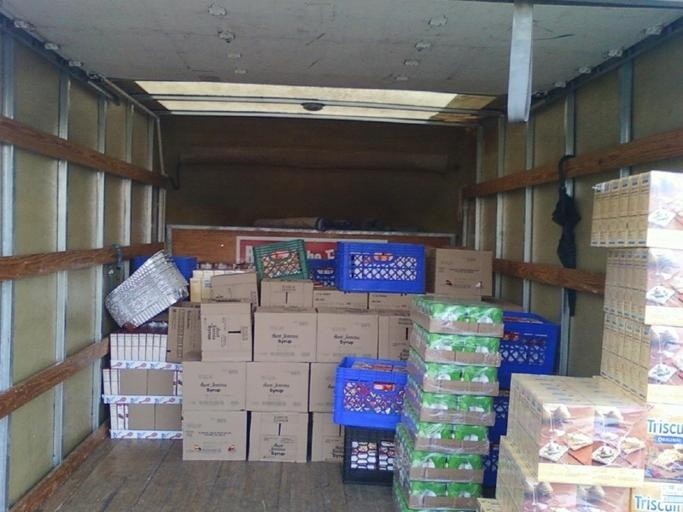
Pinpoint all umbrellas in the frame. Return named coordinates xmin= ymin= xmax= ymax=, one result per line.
xmin=551 ymin=154 xmax=581 ymax=317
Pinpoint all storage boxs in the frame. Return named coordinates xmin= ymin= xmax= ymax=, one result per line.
xmin=103 ymin=247 xmax=493 ymax=462
xmin=475 ymin=170 xmax=683 ymax=511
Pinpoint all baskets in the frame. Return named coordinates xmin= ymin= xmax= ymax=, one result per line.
xmin=483 ymin=312 xmax=560 ymax=487
xmin=332 ymin=355 xmax=406 ymax=486
xmin=252 ymin=240 xmax=426 ymax=293
